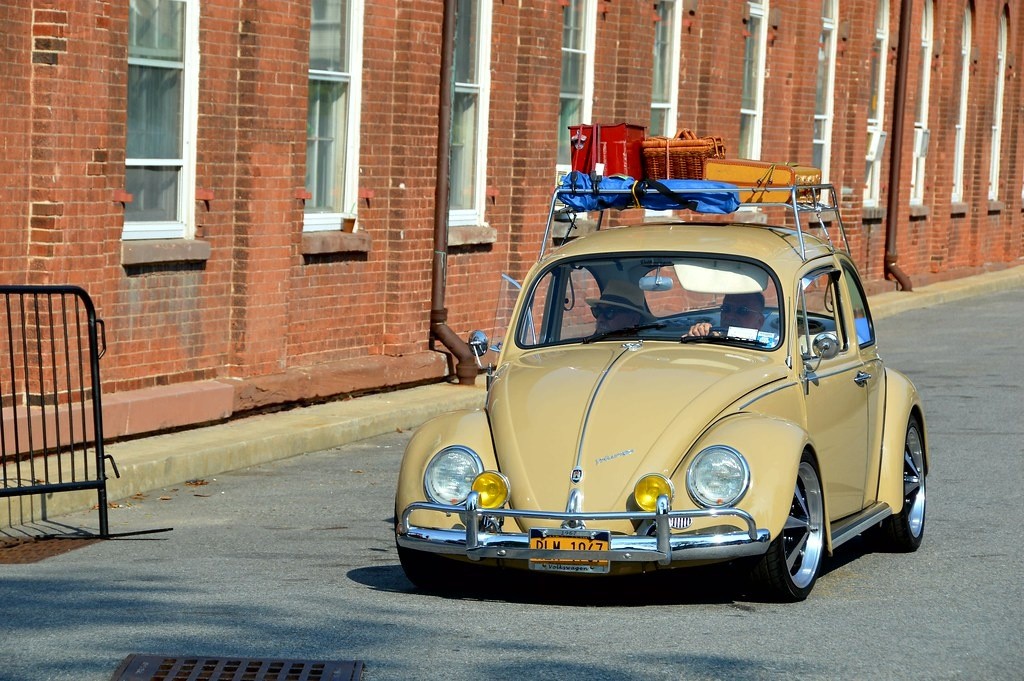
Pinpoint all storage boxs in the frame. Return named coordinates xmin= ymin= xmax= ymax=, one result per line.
xmin=641 ymin=128 xmax=727 ymax=182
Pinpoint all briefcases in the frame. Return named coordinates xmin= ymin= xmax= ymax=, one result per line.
xmin=702 ymin=157 xmax=821 ymax=205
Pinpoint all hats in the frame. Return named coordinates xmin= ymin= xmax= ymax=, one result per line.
xmin=584 ymin=277 xmax=658 ymax=322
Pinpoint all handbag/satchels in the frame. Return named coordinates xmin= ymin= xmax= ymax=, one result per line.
xmin=556 ymin=169 xmax=740 ymax=214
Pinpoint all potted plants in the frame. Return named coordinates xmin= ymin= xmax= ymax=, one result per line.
xmin=343 ymin=201 xmax=356 ymax=233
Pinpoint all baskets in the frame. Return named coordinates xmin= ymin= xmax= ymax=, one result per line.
xmin=642 ymin=126 xmax=727 ymax=179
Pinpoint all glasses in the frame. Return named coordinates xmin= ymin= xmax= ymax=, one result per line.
xmin=720 ymin=304 xmax=760 ymax=316
xmin=590 ymin=306 xmax=633 ymax=319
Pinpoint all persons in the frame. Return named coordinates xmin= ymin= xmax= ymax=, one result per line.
xmin=681 ymin=291 xmax=767 ymax=347
xmin=583 ymin=278 xmax=654 ymax=338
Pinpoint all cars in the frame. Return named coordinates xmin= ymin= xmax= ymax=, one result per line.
xmin=394 ymin=184 xmax=927 ymax=603
